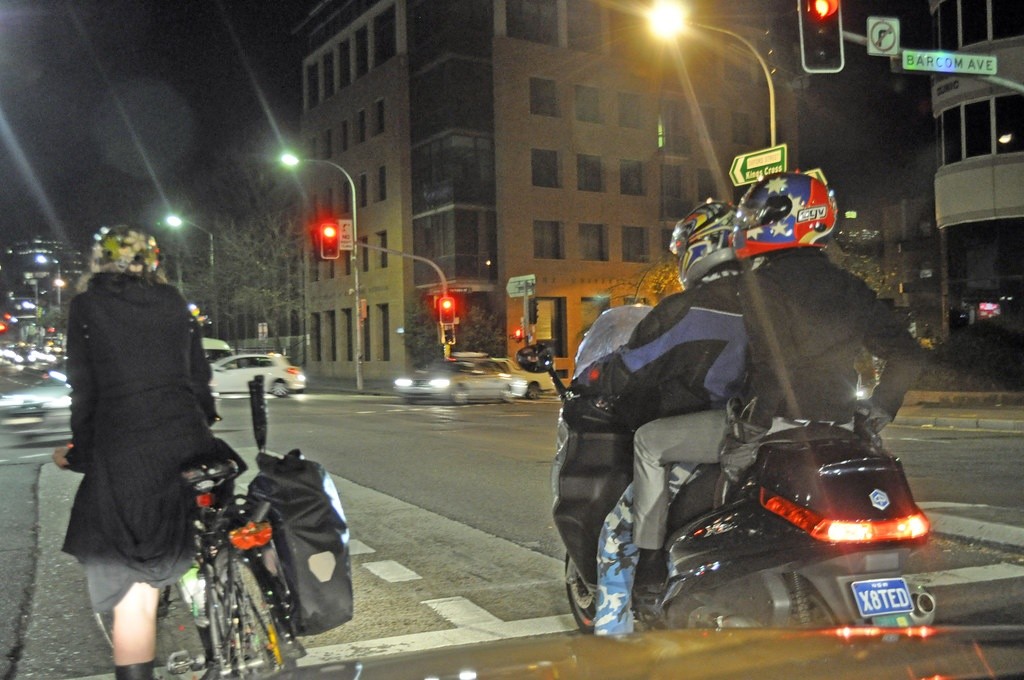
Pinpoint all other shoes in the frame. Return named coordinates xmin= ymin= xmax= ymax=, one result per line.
xmin=630 ymin=563 xmax=669 ymax=595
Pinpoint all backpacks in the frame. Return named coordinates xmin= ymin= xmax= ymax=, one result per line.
xmin=245 ymin=449 xmax=354 ymax=638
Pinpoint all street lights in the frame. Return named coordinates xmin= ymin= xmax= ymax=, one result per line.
xmin=646 ymin=0 xmax=777 ymax=148
xmin=166 ymin=216 xmax=220 ymax=340
xmin=280 ymin=154 xmax=364 ymax=390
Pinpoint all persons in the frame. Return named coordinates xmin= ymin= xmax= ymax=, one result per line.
xmin=597 ymin=203 xmax=748 ymax=637
xmin=51 ymin=225 xmax=248 ymax=680
xmin=632 ymin=172 xmax=922 ymax=585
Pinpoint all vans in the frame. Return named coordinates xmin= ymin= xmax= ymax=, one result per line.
xmin=198 ymin=336 xmax=237 ymax=368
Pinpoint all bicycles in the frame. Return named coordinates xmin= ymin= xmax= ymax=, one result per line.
xmin=63 ymin=414 xmax=308 ymax=679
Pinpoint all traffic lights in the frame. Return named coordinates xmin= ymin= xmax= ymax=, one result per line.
xmin=797 ymin=0 xmax=846 ymax=75
xmin=515 ymin=330 xmax=521 ymax=338
xmin=320 ymin=222 xmax=340 ymax=261
xmin=439 ymin=298 xmax=456 ymax=325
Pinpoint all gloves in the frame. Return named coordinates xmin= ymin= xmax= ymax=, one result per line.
xmin=716 ymin=418 xmax=769 ymax=483
xmin=855 ymin=398 xmax=893 ymax=449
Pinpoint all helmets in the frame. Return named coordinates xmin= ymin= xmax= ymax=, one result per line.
xmin=732 ymin=170 xmax=839 ymax=259
xmin=669 ymin=200 xmax=739 ymax=290
xmin=89 ymin=223 xmax=160 ymax=275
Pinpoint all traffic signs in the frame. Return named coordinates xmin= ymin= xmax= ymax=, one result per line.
xmin=728 ymin=144 xmax=788 ymax=188
xmin=803 ymin=166 xmax=829 ymax=188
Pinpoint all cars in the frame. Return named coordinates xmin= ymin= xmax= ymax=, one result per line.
xmin=393 ymin=352 xmax=556 ymax=406
xmin=208 ymin=352 xmax=308 ymax=398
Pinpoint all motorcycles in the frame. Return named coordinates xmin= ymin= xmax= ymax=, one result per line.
xmin=516 ymin=304 xmax=935 ymax=633
